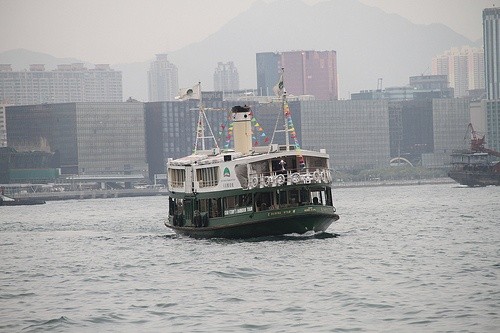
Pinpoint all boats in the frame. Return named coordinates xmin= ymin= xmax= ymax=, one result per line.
xmin=161 ymin=66 xmax=341 ymax=238
xmin=0 ymin=193 xmax=48 ymax=207
xmin=446 ymin=123 xmax=500 ymax=186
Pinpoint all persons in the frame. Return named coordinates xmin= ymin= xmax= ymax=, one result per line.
xmin=311 ymin=196 xmax=321 ymax=205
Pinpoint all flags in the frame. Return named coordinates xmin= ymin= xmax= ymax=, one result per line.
xmin=272 ymin=70 xmax=285 ymax=99
xmin=175 ymin=83 xmax=200 ymax=100
